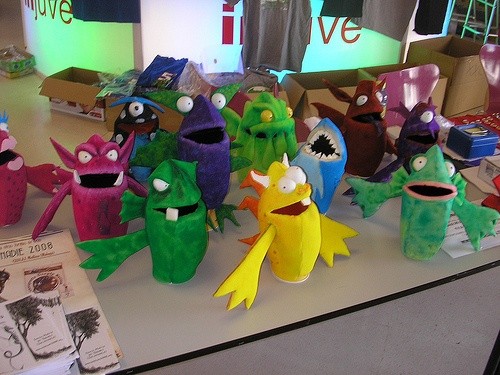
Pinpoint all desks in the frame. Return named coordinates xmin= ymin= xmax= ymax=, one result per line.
xmin=0 ymin=142 xmax=500 ymax=375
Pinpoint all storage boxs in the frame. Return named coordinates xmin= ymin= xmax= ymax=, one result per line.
xmin=0 ymin=44 xmax=35 ymax=79
xmin=38 ymin=35 xmax=499 ymax=159
